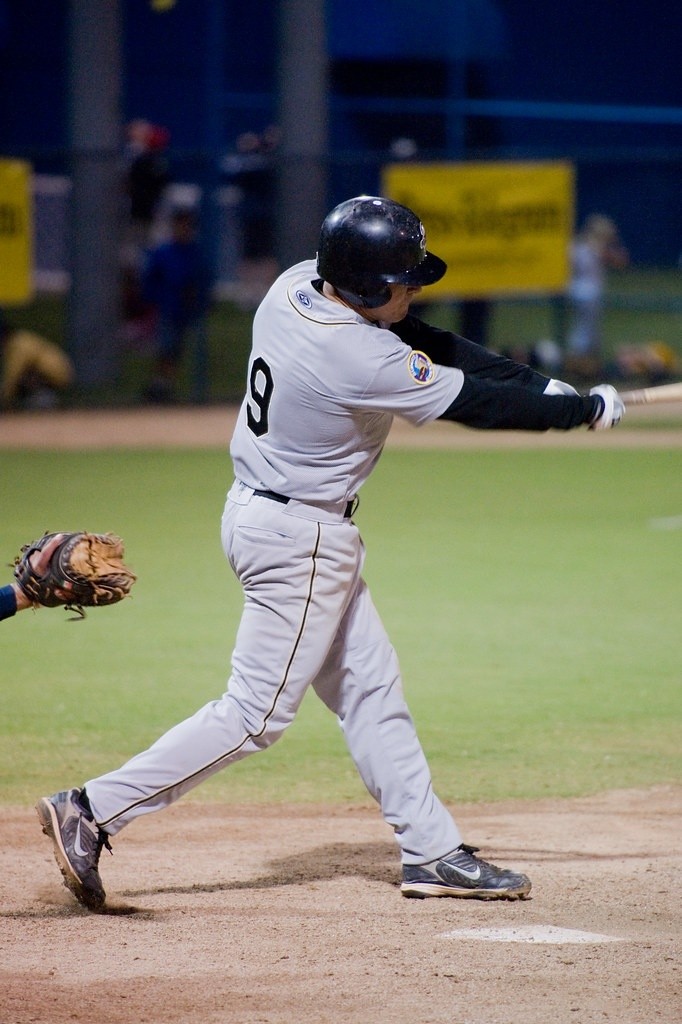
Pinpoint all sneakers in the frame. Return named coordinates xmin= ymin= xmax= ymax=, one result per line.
xmin=401 ymin=843 xmax=531 ymax=899
xmin=36 ymin=786 xmax=113 ymax=911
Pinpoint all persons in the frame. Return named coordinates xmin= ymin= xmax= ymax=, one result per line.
xmin=0 ymin=530 xmax=134 ymax=622
xmin=123 ymin=115 xmax=630 ymax=407
xmin=31 ymin=194 xmax=626 ymax=911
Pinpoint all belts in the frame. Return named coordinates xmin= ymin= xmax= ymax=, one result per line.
xmin=253 ymin=489 xmax=354 ymax=518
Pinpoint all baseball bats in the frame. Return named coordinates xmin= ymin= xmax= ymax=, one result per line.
xmin=618 ymin=382 xmax=682 ymax=412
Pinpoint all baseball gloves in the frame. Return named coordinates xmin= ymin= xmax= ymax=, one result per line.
xmin=13 ymin=522 xmax=140 ymax=616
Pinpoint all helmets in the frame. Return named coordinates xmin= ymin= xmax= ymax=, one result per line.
xmin=315 ymin=195 xmax=448 ymax=309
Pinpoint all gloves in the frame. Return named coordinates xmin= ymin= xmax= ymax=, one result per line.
xmin=543 ymin=378 xmax=581 ymax=397
xmin=588 ymin=384 xmax=625 ymax=432
